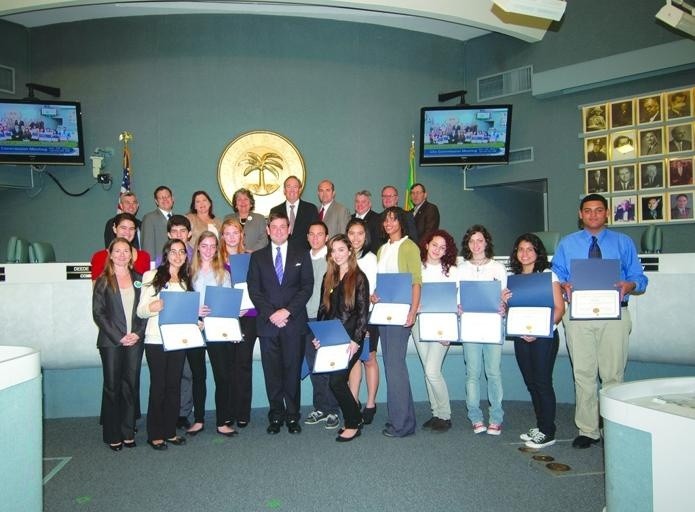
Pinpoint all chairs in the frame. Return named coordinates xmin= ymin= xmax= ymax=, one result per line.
xmin=532 ymin=231 xmax=561 ymax=255
xmin=640 ymin=225 xmax=663 ymax=256
xmin=0 ymin=236 xmax=28 ymax=263
xmin=26 ymin=241 xmax=58 ymax=263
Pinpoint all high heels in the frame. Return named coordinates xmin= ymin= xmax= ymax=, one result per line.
xmin=361 ymin=404 xmax=377 ymax=425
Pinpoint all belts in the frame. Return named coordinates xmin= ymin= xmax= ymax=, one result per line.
xmin=620 ymin=300 xmax=629 ymax=308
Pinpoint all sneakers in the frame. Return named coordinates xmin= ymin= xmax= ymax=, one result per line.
xmin=519 ymin=428 xmax=541 ymax=442
xmin=472 ymin=421 xmax=486 ymax=434
xmin=303 ymin=411 xmax=328 ymax=425
xmin=377 ymin=416 xmax=453 ymax=438
xmin=572 ymin=435 xmax=602 ymax=449
xmin=486 ymin=424 xmax=503 ymax=437
xmin=325 ymin=413 xmax=340 ymax=430
xmin=524 ymin=432 xmax=557 ymax=449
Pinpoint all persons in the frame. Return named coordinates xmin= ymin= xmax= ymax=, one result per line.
xmin=2 ymin=107 xmax=78 ymax=143
xmin=428 ymin=113 xmax=507 ymax=144
xmin=502 ymin=234 xmax=568 ymax=451
xmin=583 ymin=90 xmax=694 ymax=226
xmin=551 ymin=195 xmax=650 ymax=450
xmin=414 ymin=230 xmax=459 ymax=433
xmin=457 ymin=224 xmax=507 ymax=435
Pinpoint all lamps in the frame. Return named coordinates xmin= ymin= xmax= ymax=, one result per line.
xmin=655 ymin=0 xmax=695 ymax=36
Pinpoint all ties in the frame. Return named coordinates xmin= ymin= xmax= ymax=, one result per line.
xmin=588 ymin=236 xmax=603 ymax=260
xmin=274 ymin=247 xmax=284 ymax=286
xmin=289 ymin=205 xmax=296 ymax=235
xmin=319 ymin=207 xmax=325 ymax=221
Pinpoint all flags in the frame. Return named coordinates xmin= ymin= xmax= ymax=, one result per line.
xmin=404 ymin=149 xmax=417 ymax=211
xmin=115 ymin=153 xmax=134 ymax=215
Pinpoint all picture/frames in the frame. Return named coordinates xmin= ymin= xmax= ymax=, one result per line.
xmin=572 ymin=87 xmax=694 ymax=230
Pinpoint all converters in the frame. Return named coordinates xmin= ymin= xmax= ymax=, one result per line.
xmin=97 ymin=174 xmax=109 ymax=183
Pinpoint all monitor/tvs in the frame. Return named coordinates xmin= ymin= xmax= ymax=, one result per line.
xmin=0 ymin=99 xmax=85 ymax=165
xmin=420 ymin=104 xmax=512 ymax=165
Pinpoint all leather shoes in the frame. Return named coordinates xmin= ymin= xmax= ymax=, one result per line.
xmin=108 ymin=436 xmax=186 ymax=451
xmin=185 ymin=421 xmax=248 ymax=437
xmin=335 ymin=428 xmax=361 ymax=443
xmin=285 ymin=417 xmax=302 ymax=433
xmin=267 ymin=418 xmax=282 ymax=434
